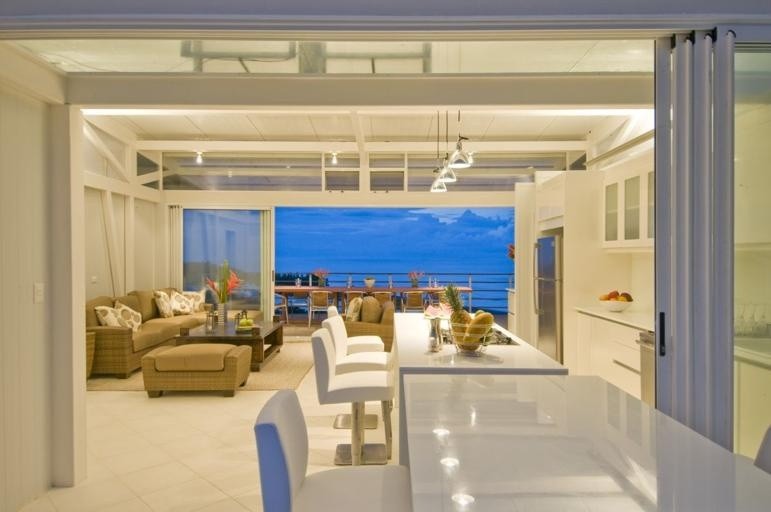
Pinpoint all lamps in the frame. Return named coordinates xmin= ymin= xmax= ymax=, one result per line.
xmin=330 ymin=154 xmax=339 ymax=164
xmin=448 ymin=142 xmax=472 ymax=169
xmin=437 ymin=159 xmax=457 ymax=184
xmin=429 ymin=177 xmax=447 ymax=192
xmin=195 ymin=152 xmax=203 ymax=164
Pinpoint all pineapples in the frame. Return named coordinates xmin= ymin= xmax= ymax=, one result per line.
xmin=443 ymin=284 xmax=472 ymax=336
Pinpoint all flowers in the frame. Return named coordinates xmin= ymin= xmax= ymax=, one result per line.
xmin=202 ymin=260 xmax=245 ymax=304
xmin=408 ymin=272 xmax=424 ymax=280
xmin=314 ymin=269 xmax=329 ymax=278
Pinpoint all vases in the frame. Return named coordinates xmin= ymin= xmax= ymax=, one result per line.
xmin=318 ymin=279 xmax=327 ymax=287
xmin=411 ymin=280 xmax=419 ymax=287
xmin=217 ymin=304 xmax=229 ymax=324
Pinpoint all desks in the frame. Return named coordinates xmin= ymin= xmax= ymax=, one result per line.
xmin=276 ymin=285 xmax=472 ymax=323
xmin=403 ymin=374 xmax=771 ymax=511
xmin=394 ymin=312 xmax=569 ymax=465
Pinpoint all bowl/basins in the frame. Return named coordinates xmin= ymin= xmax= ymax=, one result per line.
xmin=364 ymin=279 xmax=375 ymax=288
xmin=600 ymin=300 xmax=632 ymax=312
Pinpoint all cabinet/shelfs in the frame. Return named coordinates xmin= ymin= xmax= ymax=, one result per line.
xmin=595 ymin=149 xmax=656 ymax=252
xmin=572 ymin=303 xmax=655 ymax=405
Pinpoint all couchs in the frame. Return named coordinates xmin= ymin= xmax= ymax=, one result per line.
xmin=86 ymin=287 xmax=215 ymax=379
xmin=141 ymin=344 xmax=252 ymax=397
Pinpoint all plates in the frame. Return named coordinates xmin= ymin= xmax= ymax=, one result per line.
xmin=235 ymin=324 xmax=263 ymax=331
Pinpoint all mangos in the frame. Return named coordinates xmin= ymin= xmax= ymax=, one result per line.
xmin=462 ymin=312 xmax=495 ymax=347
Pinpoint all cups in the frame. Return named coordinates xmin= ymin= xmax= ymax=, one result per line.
xmin=295 ymin=279 xmax=301 ymax=288
xmin=388 ymin=275 xmax=393 ymax=289
xmin=205 ymin=315 xmax=214 ymax=335
xmin=433 ymin=277 xmax=439 ymax=288
xmin=347 ymin=276 xmax=352 ymax=288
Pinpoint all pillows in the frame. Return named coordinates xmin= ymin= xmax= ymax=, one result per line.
xmin=94 ymin=306 xmax=130 ymax=331
xmin=152 ymin=292 xmax=174 ymax=318
xmin=171 ymin=291 xmax=191 ymax=315
xmin=114 ymin=300 xmax=142 ymax=332
xmin=182 ymin=289 xmax=206 ymax=312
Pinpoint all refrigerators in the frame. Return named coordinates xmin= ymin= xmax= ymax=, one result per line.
xmin=532 ymin=234 xmax=562 ymax=364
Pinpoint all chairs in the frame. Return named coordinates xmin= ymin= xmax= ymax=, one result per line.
xmin=321 ymin=315 xmax=394 ymax=429
xmin=373 ymin=291 xmax=392 ymax=305
xmin=327 ymin=306 xmax=385 ymax=353
xmin=401 ymin=292 xmax=426 ymax=313
xmin=275 ymin=293 xmax=289 ymax=325
xmin=311 ymin=327 xmax=395 ymax=466
xmin=342 ymin=290 xmax=368 ymax=318
xmin=307 ymin=290 xmax=335 ymax=328
xmin=254 ymin=390 xmax=409 ymax=511
